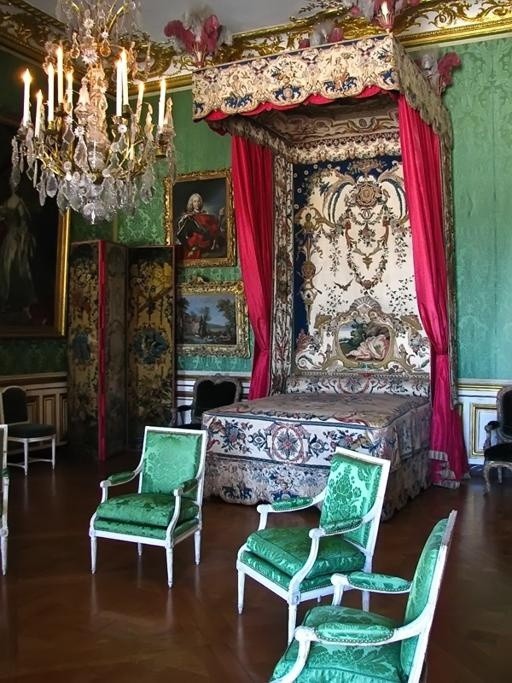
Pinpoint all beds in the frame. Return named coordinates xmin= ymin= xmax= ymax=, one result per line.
xmin=164 ymin=0 xmax=468 ymax=521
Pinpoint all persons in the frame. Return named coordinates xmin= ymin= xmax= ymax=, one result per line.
xmin=176 ymin=193 xmax=217 ymax=258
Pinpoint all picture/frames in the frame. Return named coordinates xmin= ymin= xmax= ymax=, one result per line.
xmin=0 ymin=177 xmax=73 ymax=340
xmin=175 ymin=274 xmax=253 ymax=360
xmin=162 ymin=164 xmax=238 ymax=269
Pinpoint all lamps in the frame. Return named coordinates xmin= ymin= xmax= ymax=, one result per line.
xmin=8 ymin=2 xmax=179 ymax=223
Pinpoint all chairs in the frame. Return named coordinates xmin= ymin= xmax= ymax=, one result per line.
xmin=1 ymin=424 xmax=13 ymax=573
xmin=169 ymin=373 xmax=242 ymax=431
xmin=267 ymin=507 xmax=459 ymax=682
xmin=1 ymin=383 xmax=58 ymax=476
xmin=234 ymin=446 xmax=394 ymax=648
xmin=88 ymin=424 xmax=212 ymax=590
xmin=480 ymin=383 xmax=512 ymax=497
